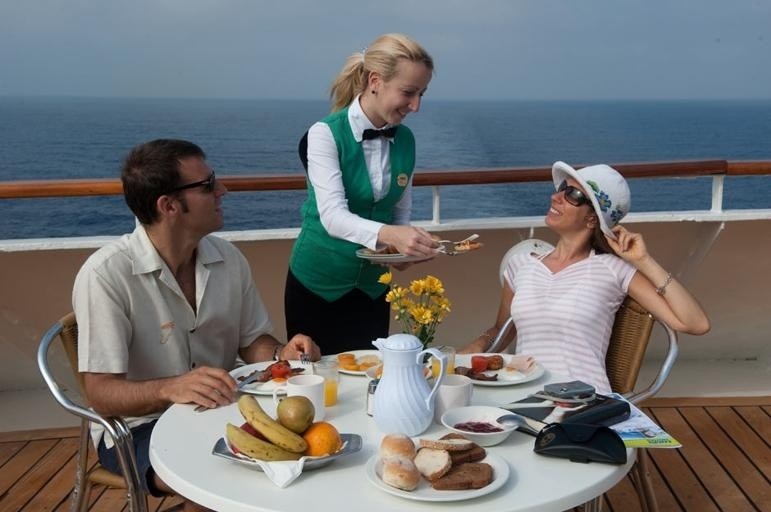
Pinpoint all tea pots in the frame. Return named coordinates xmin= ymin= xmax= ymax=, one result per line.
xmin=371 ymin=335 xmax=445 ymax=436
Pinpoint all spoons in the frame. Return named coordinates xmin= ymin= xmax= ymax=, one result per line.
xmin=494 ymin=412 xmax=541 ymax=433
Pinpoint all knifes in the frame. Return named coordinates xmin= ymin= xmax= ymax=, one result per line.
xmin=197 ymin=369 xmax=266 ymax=411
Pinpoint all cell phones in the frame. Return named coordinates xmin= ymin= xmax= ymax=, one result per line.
xmin=544 ymin=380 xmax=595 ymax=399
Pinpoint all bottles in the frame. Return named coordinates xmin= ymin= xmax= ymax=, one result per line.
xmin=366 ymin=379 xmax=381 ymax=416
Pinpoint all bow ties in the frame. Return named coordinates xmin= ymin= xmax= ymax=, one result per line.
xmin=361 ymin=127 xmax=397 ymax=141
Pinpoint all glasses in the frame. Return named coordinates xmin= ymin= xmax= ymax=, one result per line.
xmin=558 ymin=180 xmax=593 ymax=209
xmin=178 ymin=170 xmax=215 ymax=192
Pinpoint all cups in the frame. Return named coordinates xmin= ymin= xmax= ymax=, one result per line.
xmin=435 ymin=373 xmax=472 ymax=425
xmin=273 ymin=374 xmax=327 ymax=419
xmin=434 ymin=344 xmax=456 ymax=377
xmin=314 ymin=358 xmax=341 ymax=405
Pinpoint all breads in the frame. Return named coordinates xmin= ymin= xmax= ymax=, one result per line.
xmin=380 ymin=433 xmax=415 ymax=462
xmin=383 ymin=457 xmax=420 ymax=490
xmin=414 ymin=432 xmax=492 ymax=490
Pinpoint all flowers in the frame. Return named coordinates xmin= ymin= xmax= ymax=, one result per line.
xmin=377 ymin=272 xmax=451 ymax=363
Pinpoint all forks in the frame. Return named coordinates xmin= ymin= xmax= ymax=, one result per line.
xmin=440 ymin=233 xmax=480 ymax=244
xmin=300 ymin=351 xmax=311 ymax=364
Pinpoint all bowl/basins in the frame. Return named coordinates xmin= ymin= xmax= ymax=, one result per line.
xmin=441 ymin=405 xmax=518 ymax=446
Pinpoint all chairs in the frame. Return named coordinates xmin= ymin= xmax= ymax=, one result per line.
xmin=37 ymin=311 xmax=248 ymax=512
xmin=484 ymin=295 xmax=680 ymax=512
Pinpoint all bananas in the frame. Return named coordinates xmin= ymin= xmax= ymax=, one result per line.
xmin=227 ymin=423 xmax=303 ymax=461
xmin=238 ymin=395 xmax=308 ymax=453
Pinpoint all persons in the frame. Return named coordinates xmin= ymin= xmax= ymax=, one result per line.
xmin=284 ymin=32 xmax=442 ymax=356
xmin=453 ymin=159 xmax=711 ymax=402
xmin=70 ymin=139 xmax=321 ymax=512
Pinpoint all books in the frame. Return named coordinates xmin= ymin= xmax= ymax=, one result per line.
xmin=498 ymin=390 xmax=682 ymax=450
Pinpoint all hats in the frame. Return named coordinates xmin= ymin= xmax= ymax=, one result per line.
xmin=552 ymin=161 xmax=632 ymax=242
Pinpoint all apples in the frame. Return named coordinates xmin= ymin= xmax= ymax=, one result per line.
xmin=277 ymin=396 xmax=314 ymax=433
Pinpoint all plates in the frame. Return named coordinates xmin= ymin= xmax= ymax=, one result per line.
xmin=356 ymin=247 xmax=439 ymax=263
xmin=454 ymin=351 xmax=544 ymax=385
xmin=364 ymin=447 xmax=513 ymax=503
xmin=211 ymin=429 xmax=364 ymax=470
xmin=365 ymin=364 xmax=433 ymax=383
xmin=335 ymin=349 xmax=383 ymax=374
xmin=229 ymin=360 xmax=322 ymax=396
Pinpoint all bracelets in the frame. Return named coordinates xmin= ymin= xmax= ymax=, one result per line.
xmin=656 ymin=272 xmax=673 ymax=296
xmin=273 ymin=344 xmax=286 ymax=362
xmin=479 ymin=332 xmax=493 ymax=346
